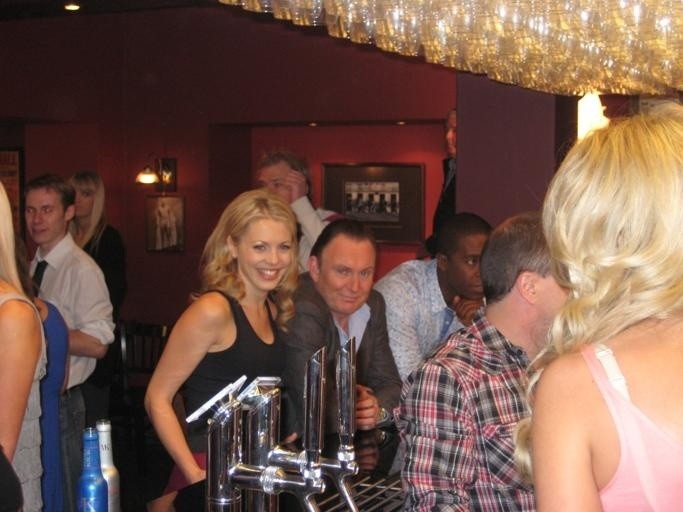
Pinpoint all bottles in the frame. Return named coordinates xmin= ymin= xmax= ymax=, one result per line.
xmin=94 ymin=419 xmax=121 ymax=510
xmin=74 ymin=427 xmax=108 ymax=511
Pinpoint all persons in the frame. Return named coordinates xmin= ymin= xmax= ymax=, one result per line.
xmin=66 ymin=171 xmax=126 ymax=428
xmin=254 ymin=151 xmax=346 ymax=275
xmin=416 ymin=109 xmax=457 ymax=261
xmin=143 ymin=187 xmax=298 ymax=512
xmin=392 ymin=210 xmax=573 ymax=512
xmin=15 ymin=236 xmax=71 ymax=512
xmin=354 ymin=426 xmax=397 ymax=472
xmin=0 ymin=180 xmax=48 ymax=512
xmin=512 ymin=99 xmax=683 ymax=512
xmin=371 ymin=212 xmax=493 ymax=384
xmin=283 ymin=219 xmax=404 ymax=438
xmin=23 ymin=174 xmax=117 ymax=512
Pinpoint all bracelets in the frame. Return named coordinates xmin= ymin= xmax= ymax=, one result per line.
xmin=375 ymin=428 xmax=385 ymax=443
xmin=376 ymin=408 xmax=386 ymax=422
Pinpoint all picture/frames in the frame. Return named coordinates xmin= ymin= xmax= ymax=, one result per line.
xmin=321 ymin=162 xmax=425 ymax=246
xmin=142 ymin=194 xmax=187 ymax=255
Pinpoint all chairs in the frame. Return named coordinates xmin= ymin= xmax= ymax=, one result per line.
xmin=110 ymin=319 xmax=171 ymax=479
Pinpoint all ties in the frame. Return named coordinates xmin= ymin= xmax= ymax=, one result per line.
xmin=31 ymin=260 xmax=48 ymax=298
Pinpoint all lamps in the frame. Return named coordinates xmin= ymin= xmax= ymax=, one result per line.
xmin=137 ymin=157 xmax=178 ymax=193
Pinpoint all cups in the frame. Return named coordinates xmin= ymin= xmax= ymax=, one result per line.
xmin=215 ymin=1 xmax=681 ymax=99
